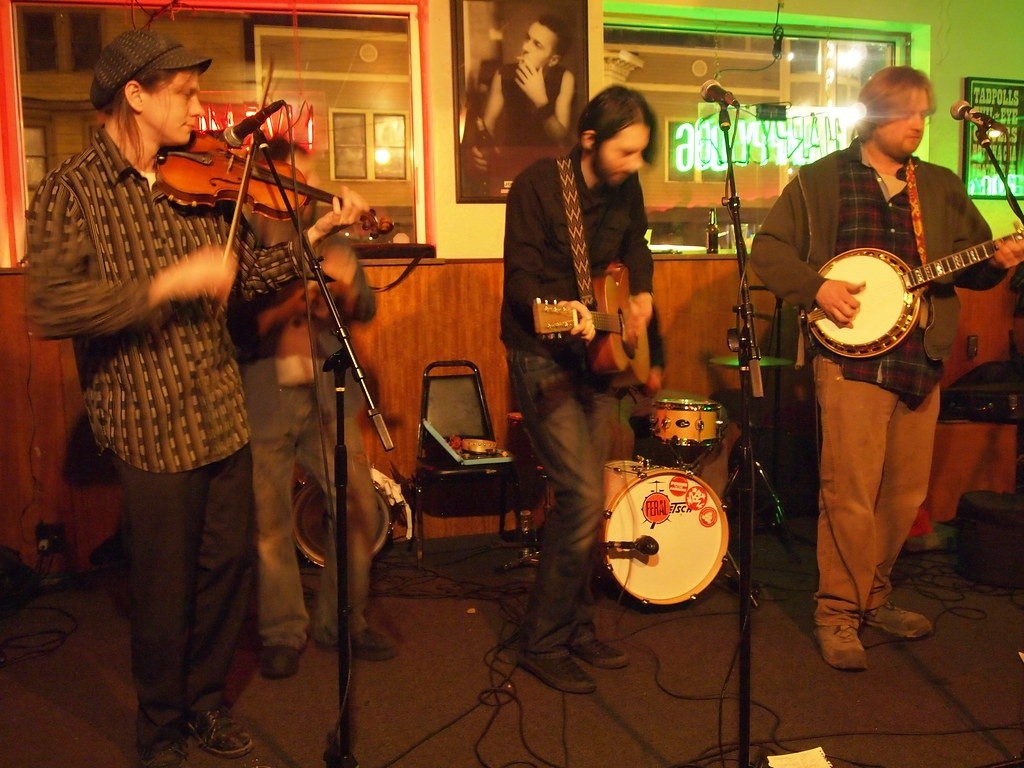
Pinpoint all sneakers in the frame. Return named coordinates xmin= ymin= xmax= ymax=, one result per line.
xmin=570 ymin=640 xmax=629 ymax=669
xmin=516 ymin=651 xmax=595 ymax=694
xmin=864 ymin=602 xmax=931 ymax=638
xmin=184 ymin=708 xmax=253 ymax=759
xmin=137 ymin=744 xmax=184 ymax=768
xmin=810 ymin=625 xmax=866 ymax=671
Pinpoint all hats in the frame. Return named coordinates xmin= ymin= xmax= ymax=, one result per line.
xmin=89 ymin=31 xmax=213 ymax=108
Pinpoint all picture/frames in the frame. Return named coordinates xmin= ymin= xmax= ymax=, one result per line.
xmin=449 ymin=0 xmax=588 ymax=204
xmin=961 ymin=77 xmax=1024 ymax=201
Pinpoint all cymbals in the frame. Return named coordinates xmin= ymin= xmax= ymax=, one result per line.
xmin=709 ymin=357 xmax=795 ymax=367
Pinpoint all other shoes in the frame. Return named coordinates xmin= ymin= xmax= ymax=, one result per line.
xmin=316 ymin=630 xmax=393 ymax=662
xmin=262 ymin=646 xmax=299 ymax=676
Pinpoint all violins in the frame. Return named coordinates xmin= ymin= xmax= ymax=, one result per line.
xmin=156 ymin=129 xmax=396 ymax=239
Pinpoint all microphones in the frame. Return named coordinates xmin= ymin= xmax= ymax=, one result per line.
xmin=635 ymin=535 xmax=659 ymax=555
xmin=224 ymin=98 xmax=284 ymax=148
xmin=950 ymin=100 xmax=1008 ymax=133
xmin=700 ymin=80 xmax=741 ymax=109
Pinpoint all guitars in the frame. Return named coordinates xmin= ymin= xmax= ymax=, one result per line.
xmin=805 ymin=220 xmax=1024 ymax=358
xmin=531 ymin=260 xmax=650 ymax=389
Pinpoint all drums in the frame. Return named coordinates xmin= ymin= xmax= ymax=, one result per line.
xmin=601 ymin=460 xmax=729 ymax=606
xmin=651 ymin=398 xmax=724 ymax=446
xmin=292 ymin=477 xmax=392 ymax=569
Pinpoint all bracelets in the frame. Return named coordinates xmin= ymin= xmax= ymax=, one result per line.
xmin=536 ymin=102 xmax=555 ymax=120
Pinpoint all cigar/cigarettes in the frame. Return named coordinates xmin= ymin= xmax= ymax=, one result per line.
xmin=516 ymin=57 xmax=525 ymax=60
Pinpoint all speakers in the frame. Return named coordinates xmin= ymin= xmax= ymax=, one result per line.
xmin=924 ymin=420 xmax=1018 ymax=523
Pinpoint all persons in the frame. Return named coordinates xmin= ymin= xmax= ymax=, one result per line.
xmin=499 ymin=85 xmax=657 ymax=693
xmin=471 ymin=14 xmax=575 ymax=180
xmin=23 ymin=28 xmax=370 ymax=768
xmin=606 ymin=302 xmax=728 ymax=500
xmin=749 ymin=66 xmax=1024 ymax=670
xmin=227 ymin=149 xmax=401 ymax=679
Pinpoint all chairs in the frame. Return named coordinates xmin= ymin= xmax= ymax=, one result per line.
xmin=404 ymin=360 xmax=544 ymax=573
